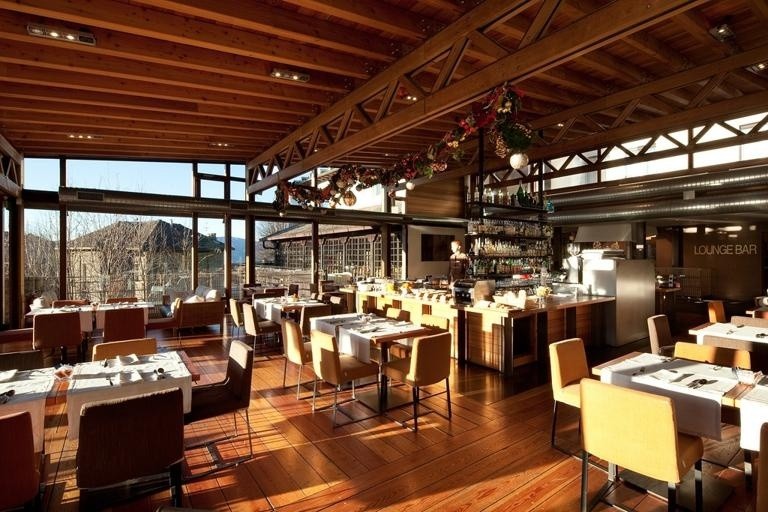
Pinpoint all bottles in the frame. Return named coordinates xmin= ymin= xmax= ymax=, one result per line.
xmin=468 ymin=237 xmax=548 ymax=258
xmin=467 ymin=178 xmax=554 ymax=212
xmin=472 ymin=257 xmax=543 ymax=275
xmin=467 ymin=218 xmax=542 ymax=236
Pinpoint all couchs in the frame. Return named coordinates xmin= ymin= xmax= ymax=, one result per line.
xmin=162 ymin=286 xmax=228 ymax=337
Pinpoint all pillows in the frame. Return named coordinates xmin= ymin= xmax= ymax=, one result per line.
xmin=170 ymin=298 xmax=176 ymax=317
xmin=195 ymin=285 xmax=205 ymax=295
xmin=184 ymin=295 xmax=204 ymax=302
xmin=206 ymin=288 xmax=222 ymax=302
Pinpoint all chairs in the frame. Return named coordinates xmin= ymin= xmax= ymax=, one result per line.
xmin=289 ymin=284 xmax=298 ymax=297
xmin=673 ymin=342 xmax=754 ymax=495
xmin=265 ymin=287 xmax=284 ymax=296
xmin=309 ymin=284 xmax=318 ymax=296
xmin=103 ymin=308 xmax=145 ymax=344
xmin=104 ymin=298 xmax=138 ymax=304
xmin=373 ymin=306 xmax=411 ymax=388
xmin=241 ymin=304 xmax=282 ymax=357
xmin=53 ymin=299 xmax=88 ymax=307
xmin=647 ymin=314 xmax=676 ymax=358
xmin=577 ymin=377 xmax=704 ymax=510
xmin=242 ymin=283 xmax=261 ymax=288
xmin=311 ymin=293 xmax=316 ymax=300
xmin=319 ymin=280 xmax=334 ymax=288
xmin=322 ymin=285 xmax=339 ymax=291
xmin=178 ymin=339 xmax=257 ymax=460
xmin=387 ymin=313 xmax=450 ymax=396
xmin=75 ymin=387 xmax=183 ymax=512
xmin=1 ymin=410 xmax=44 ymax=509
xmin=30 ymin=310 xmax=86 ymax=365
xmin=251 ymin=293 xmax=269 ymax=305
xmin=317 ymin=295 xmax=323 ymax=302
xmin=382 ymin=332 xmax=455 ymax=430
xmin=279 ymin=316 xmax=324 ymax=400
xmin=230 ymin=298 xmax=264 ymax=340
xmin=92 ymin=337 xmax=157 ymax=361
xmin=708 ymin=301 xmax=727 ymax=323
xmin=546 ymin=338 xmax=608 ymax=473
xmin=330 ymin=296 xmax=345 ymax=315
xmin=310 ymin=330 xmax=380 ymax=427
xmin=755 ymin=310 xmax=768 ymax=320
xmin=299 ymin=304 xmax=331 ymax=336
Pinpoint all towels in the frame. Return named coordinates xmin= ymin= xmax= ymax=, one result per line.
xmin=0 ymin=368 xmax=19 ymax=382
xmin=116 ymin=369 xmax=144 ymax=387
xmin=648 ymin=367 xmax=682 ymax=385
xmin=117 ymin=353 xmax=140 ymax=366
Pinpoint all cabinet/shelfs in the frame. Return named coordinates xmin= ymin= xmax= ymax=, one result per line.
xmin=451 ymin=291 xmax=616 ymax=378
